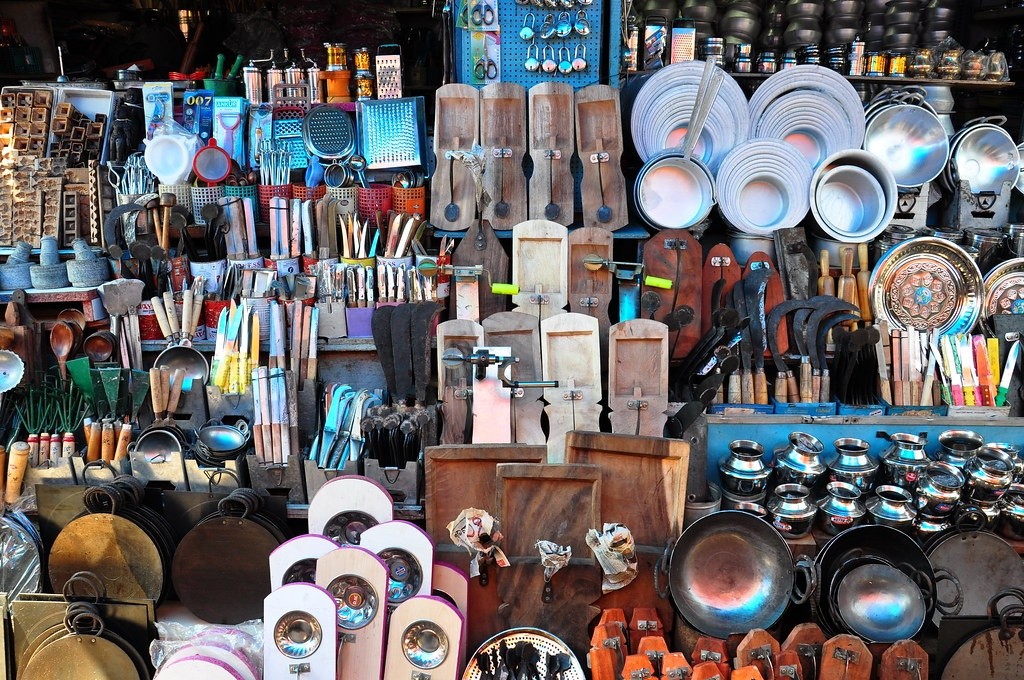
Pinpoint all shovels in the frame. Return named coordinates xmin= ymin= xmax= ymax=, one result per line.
xmin=64 ymin=277 xmax=185 ymax=464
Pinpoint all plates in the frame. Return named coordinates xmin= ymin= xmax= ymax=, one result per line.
xmin=869 ymin=235 xmax=1024 ymax=347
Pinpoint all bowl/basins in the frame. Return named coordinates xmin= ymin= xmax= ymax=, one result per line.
xmin=626 ymin=56 xmax=1020 ymax=244
xmin=191 ymin=417 xmax=251 ymax=468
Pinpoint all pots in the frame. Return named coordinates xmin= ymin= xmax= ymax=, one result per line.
xmin=649 ymin=504 xmax=1024 ymax=645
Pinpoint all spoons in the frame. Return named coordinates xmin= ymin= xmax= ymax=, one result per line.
xmin=49 ymin=304 xmax=114 ymax=391
xmin=443 ymin=145 xmax=619 ymax=251
xmin=349 ymin=151 xmax=423 ymax=222
